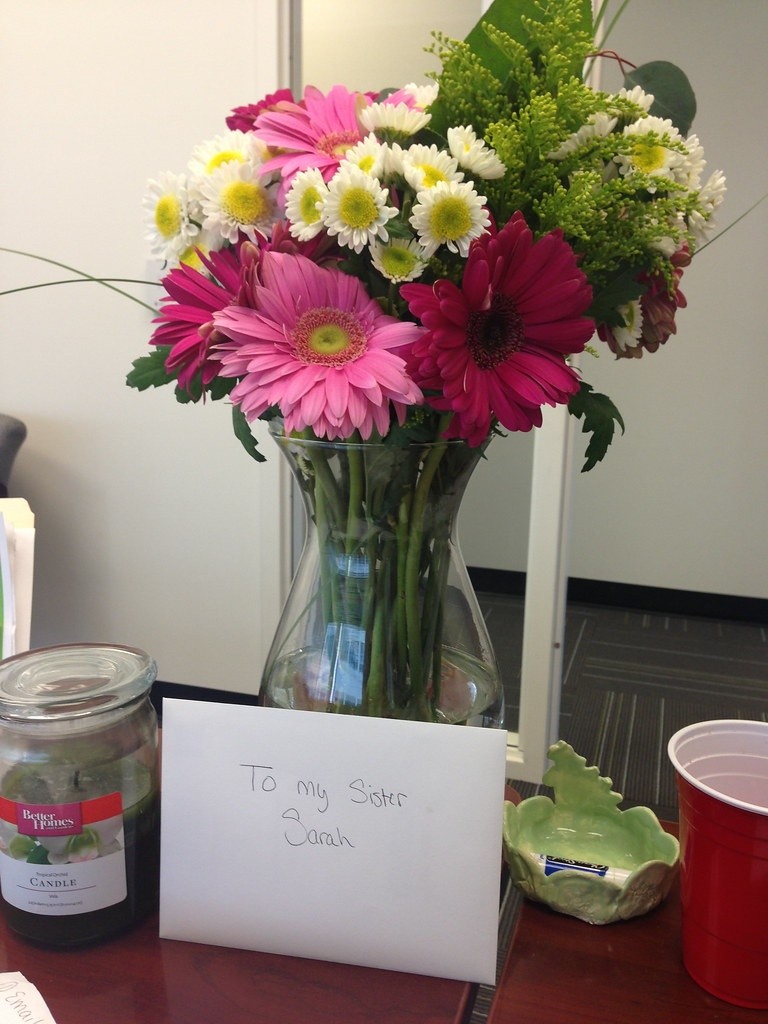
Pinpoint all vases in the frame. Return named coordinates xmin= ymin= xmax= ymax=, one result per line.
xmin=257 ymin=427 xmax=519 ymax=728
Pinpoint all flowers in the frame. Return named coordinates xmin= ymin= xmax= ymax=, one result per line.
xmin=0 ymin=0 xmax=768 ymax=725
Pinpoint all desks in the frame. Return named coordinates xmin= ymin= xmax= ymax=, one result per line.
xmin=0 ymin=723 xmax=529 ymax=1024
xmin=487 ymin=803 xmax=768 ymax=1024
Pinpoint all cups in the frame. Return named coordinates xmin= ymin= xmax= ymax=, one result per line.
xmin=666 ymin=717 xmax=768 ymax=1011
